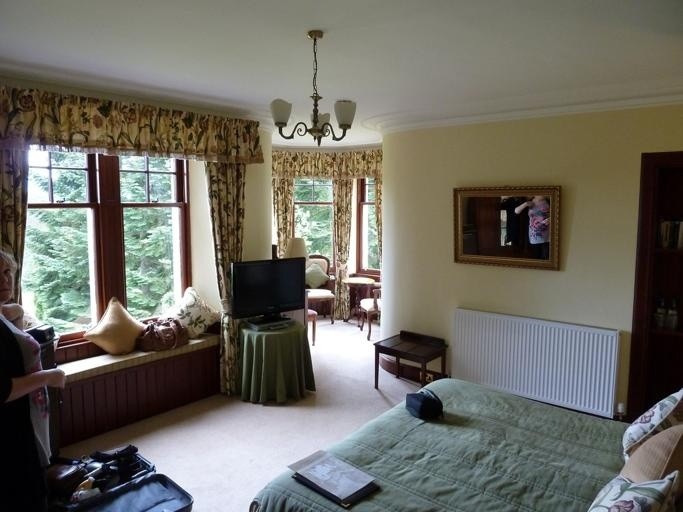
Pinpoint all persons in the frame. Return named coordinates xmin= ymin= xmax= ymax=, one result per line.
xmin=0 ymin=242 xmax=64 ymax=511
xmin=498 ymin=197 xmax=524 ymax=247
xmin=514 ymin=195 xmax=550 ymax=259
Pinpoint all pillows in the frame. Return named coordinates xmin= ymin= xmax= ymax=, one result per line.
xmin=587 ymin=469 xmax=681 ymax=512
xmin=618 ymin=425 xmax=683 ymax=484
xmin=167 ymin=286 xmax=222 ymax=339
xmin=622 ymin=388 xmax=683 ymax=463
xmin=305 ymin=264 xmax=330 ymax=289
xmin=83 ymin=297 xmax=145 ymax=355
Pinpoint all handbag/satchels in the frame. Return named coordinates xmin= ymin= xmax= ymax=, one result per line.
xmin=137 ymin=318 xmax=189 ymax=352
xmin=406 ymin=390 xmax=443 ymax=420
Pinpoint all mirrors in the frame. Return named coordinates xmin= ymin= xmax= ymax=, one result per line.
xmin=453 ymin=186 xmax=561 ymax=271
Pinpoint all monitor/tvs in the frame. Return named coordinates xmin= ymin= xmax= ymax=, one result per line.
xmin=229 ymin=255 xmax=306 ymax=324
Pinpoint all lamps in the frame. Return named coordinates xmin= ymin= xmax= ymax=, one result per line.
xmin=283 ymin=238 xmax=309 ymax=261
xmin=269 ymin=29 xmax=356 ymax=146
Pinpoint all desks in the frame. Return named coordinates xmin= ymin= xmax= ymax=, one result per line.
xmin=342 ymin=276 xmax=375 ymax=327
xmin=239 ymin=321 xmax=316 ymax=406
xmin=373 ymin=333 xmax=446 ymax=390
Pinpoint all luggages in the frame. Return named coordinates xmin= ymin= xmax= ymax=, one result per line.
xmin=47 ymin=448 xmax=194 ymax=512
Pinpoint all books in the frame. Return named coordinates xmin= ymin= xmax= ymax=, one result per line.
xmin=287 ymin=449 xmax=381 ymax=509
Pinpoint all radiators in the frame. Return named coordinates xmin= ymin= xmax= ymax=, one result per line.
xmin=452 ymin=307 xmax=625 ymax=420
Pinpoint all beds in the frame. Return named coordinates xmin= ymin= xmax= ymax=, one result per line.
xmin=250 ymin=379 xmax=683 ymax=512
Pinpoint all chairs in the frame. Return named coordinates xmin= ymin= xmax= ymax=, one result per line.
xmin=360 ymin=284 xmax=381 ymax=340
xmin=307 ymin=309 xmax=317 ymax=346
xmin=305 ymin=255 xmax=335 ymax=324
